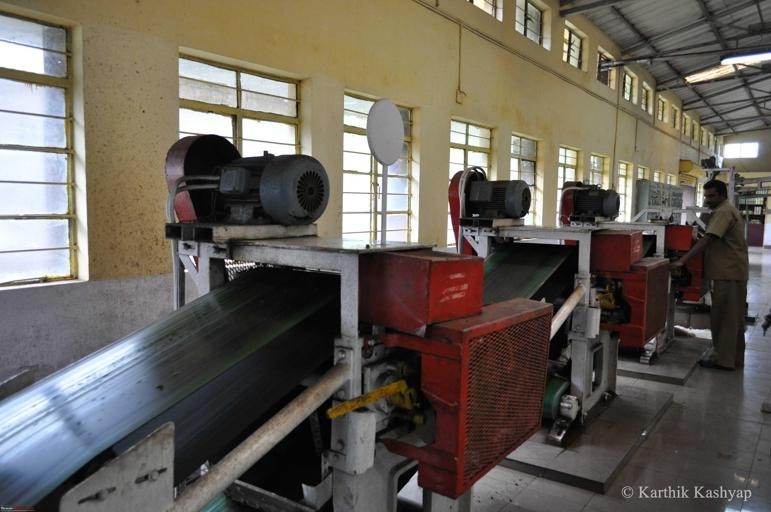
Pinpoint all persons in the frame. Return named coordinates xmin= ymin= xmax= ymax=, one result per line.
xmin=674 ymin=178 xmax=751 ymax=372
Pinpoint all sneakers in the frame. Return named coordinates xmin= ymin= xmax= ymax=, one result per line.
xmin=698 ymin=355 xmax=745 ymax=371
